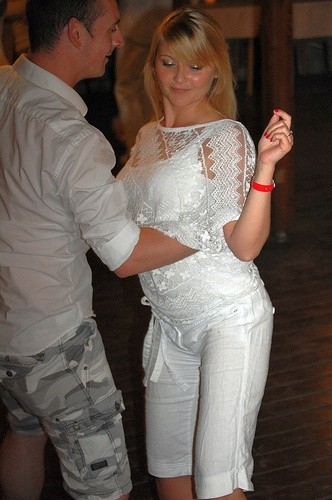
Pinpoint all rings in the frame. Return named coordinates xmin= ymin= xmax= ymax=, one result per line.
xmin=287 ymin=131 xmax=292 ymax=137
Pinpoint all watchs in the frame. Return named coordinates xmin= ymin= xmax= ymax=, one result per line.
xmin=251 ymin=176 xmax=276 ymax=192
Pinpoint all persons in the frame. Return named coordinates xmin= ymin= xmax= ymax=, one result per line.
xmin=116 ymin=8 xmax=294 ymax=500
xmin=0 ymin=0 xmax=208 ymax=500
